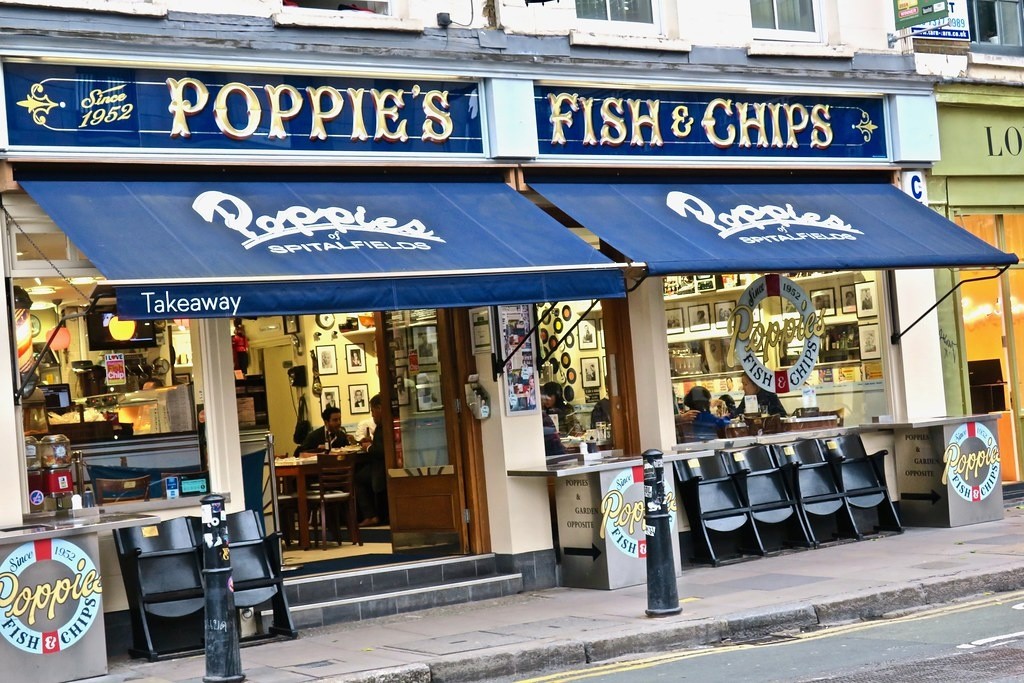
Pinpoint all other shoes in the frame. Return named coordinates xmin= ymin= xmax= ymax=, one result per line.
xmin=359 ymin=516 xmax=378 ymax=526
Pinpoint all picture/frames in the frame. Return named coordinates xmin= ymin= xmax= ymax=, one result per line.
xmin=345 ymin=342 xmax=367 ymax=373
xmin=348 ymin=383 xmax=369 ymax=414
xmin=391 ymin=325 xmax=447 ymax=413
xmin=316 ymin=345 xmax=337 ymax=375
xmin=319 ymin=386 xmax=341 ymax=418
xmin=578 ymin=270 xmax=885 ymax=388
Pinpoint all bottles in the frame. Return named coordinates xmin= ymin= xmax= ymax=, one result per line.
xmin=605 ymin=423 xmax=612 ymax=430
xmin=82 ymin=487 xmax=93 ymax=508
xmin=70 ymin=494 xmax=83 ymax=509
xmin=324 ymin=431 xmax=331 ymax=454
xmin=366 ymin=426 xmax=371 ymax=439
xmin=819 ymin=331 xmax=850 ymax=351
xmin=579 ymin=436 xmax=597 ymax=453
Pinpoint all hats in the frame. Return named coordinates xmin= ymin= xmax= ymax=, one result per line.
xmin=540 ymin=381 xmax=563 ymax=398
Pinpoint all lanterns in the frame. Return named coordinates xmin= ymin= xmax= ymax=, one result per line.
xmin=46 ymin=326 xmax=70 ymax=351
xmin=108 ymin=316 xmax=135 ymax=341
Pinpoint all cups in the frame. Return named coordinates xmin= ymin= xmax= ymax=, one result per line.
xmin=760 ymin=405 xmax=768 ymax=413
xmin=596 ymin=422 xmax=606 ymax=440
xmin=587 ymin=431 xmax=596 ymax=441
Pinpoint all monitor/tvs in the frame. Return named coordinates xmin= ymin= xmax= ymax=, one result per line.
xmin=38 ymin=384 xmax=71 ymax=407
xmin=87 ymin=310 xmax=157 ymax=351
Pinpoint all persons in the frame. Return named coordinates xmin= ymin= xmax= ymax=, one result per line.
xmin=583 ymin=325 xmax=592 ymax=343
xmin=864 ymin=333 xmax=876 ymax=352
xmin=845 ymin=292 xmax=856 ymax=306
xmin=591 ymin=375 xmax=615 ymax=451
xmin=301 ymin=408 xmax=349 ymax=452
xmin=585 ymin=364 xmax=596 ymax=381
xmin=726 ymin=377 xmax=738 ymax=392
xmin=673 ymin=372 xmax=788 ymax=443
xmin=506 ymin=360 xmax=536 ymax=411
xmin=665 ymin=307 xmax=734 ymax=328
xmin=232 ymin=325 xmax=248 ymax=374
xmin=862 ymin=289 xmax=872 ymax=310
xmin=356 ymin=394 xmax=386 ymax=529
xmin=540 ymin=382 xmax=582 ymax=465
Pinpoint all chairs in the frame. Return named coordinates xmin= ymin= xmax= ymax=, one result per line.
xmin=306 ymin=452 xmax=364 ymax=550
xmin=112 ymin=510 xmax=299 ymax=664
xmin=558 ymin=404 xmax=904 ymax=568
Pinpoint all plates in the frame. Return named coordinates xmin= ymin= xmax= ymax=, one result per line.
xmin=332 ymin=448 xmax=360 ymax=452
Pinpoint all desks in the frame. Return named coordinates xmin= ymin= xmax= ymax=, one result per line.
xmin=273 ymin=443 xmax=382 ymax=550
xmin=729 ymin=412 xmax=839 ymax=437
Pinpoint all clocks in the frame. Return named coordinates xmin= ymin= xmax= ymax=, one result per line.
xmin=315 ymin=314 xmax=336 ymax=330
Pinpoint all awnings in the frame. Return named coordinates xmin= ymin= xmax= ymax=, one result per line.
xmin=527 ymin=184 xmax=1020 ymax=345
xmin=0 ymin=182 xmax=628 ymax=405
